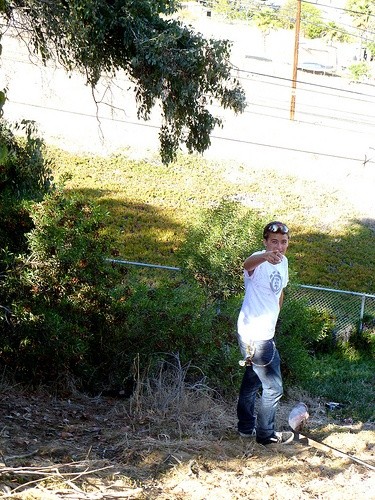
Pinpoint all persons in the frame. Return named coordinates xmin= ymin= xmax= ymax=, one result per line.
xmin=236 ymin=221 xmax=294 ymax=444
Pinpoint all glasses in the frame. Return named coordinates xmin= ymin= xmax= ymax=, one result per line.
xmin=264 ymin=224 xmax=289 ymax=238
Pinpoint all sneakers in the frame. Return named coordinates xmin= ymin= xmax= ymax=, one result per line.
xmin=239 ymin=427 xmax=257 ymax=437
xmin=260 ymin=432 xmax=294 ymax=447
